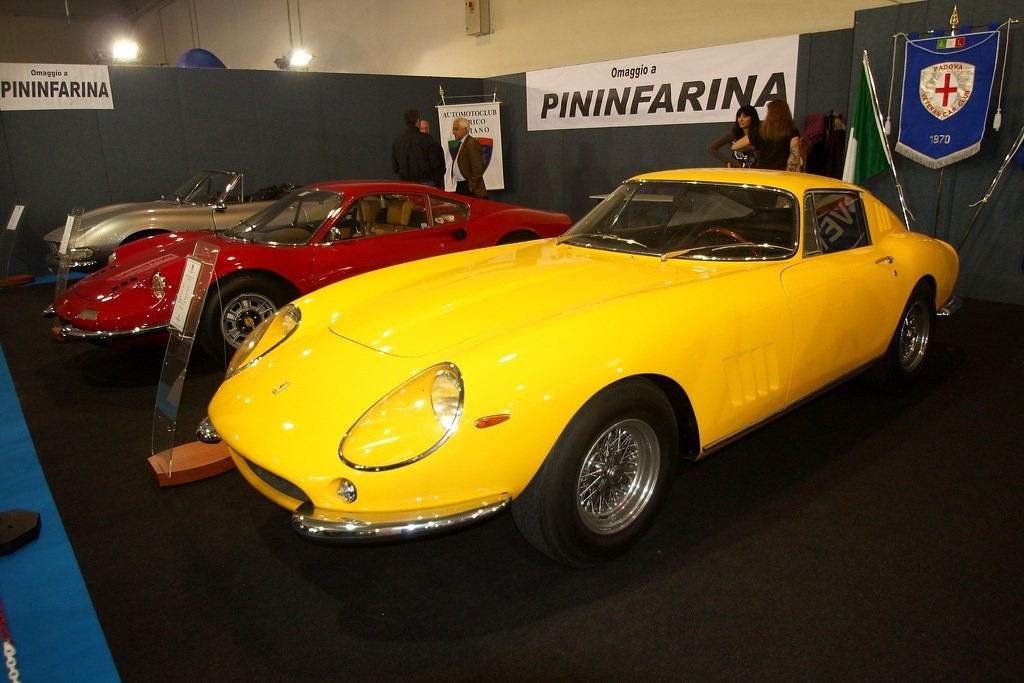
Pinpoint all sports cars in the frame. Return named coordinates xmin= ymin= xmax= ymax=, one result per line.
xmin=194 ymin=167 xmax=962 ymax=573
xmin=43 ymin=167 xmax=359 ymax=277
xmin=41 ymin=178 xmax=576 ymax=372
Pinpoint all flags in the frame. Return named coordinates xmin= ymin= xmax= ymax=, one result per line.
xmin=842 ymin=58 xmax=890 ymax=204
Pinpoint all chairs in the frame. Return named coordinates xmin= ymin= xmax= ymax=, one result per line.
xmin=249 ymin=190 xmax=267 ymax=201
xmin=371 ymin=200 xmax=420 ymax=235
xmin=354 ymin=196 xmax=381 ymax=235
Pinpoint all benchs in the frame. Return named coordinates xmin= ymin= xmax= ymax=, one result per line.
xmin=697 ymin=223 xmax=861 ymax=256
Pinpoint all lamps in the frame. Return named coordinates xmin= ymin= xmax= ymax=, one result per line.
xmin=273 ymin=49 xmax=315 ymax=71
xmin=93 ymin=38 xmax=144 ymax=64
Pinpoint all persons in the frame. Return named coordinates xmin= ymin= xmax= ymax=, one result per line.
xmin=451 ymin=118 xmax=487 ymax=198
xmin=392 ymin=109 xmax=446 ymax=188
xmin=708 ymin=100 xmax=801 ymax=173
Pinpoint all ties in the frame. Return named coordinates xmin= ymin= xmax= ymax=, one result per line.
xmin=451 ymin=141 xmax=462 ymax=185
